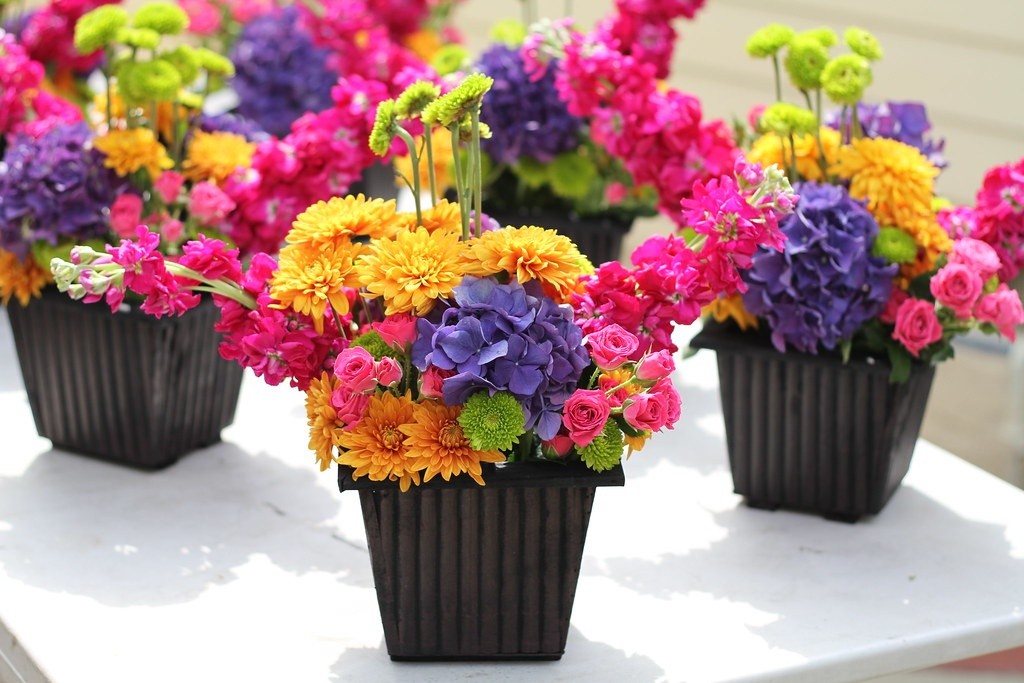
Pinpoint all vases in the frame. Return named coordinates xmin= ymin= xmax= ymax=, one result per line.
xmin=7 ymin=281 xmax=246 ymax=474
xmin=445 ymin=188 xmax=626 ymax=267
xmin=690 ymin=314 xmax=936 ymax=521
xmin=338 ymin=443 xmax=624 ymax=663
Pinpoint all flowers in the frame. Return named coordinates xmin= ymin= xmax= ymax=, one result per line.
xmin=1 ymin=1 xmax=1023 ymax=488
